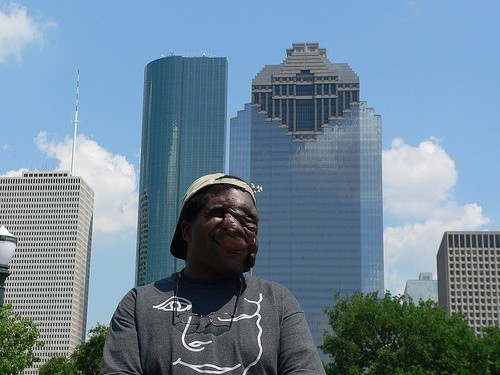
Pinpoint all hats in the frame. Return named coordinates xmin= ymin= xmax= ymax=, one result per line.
xmin=170 ymin=173 xmax=256 ymax=260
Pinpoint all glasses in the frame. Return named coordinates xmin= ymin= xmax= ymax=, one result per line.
xmin=175 ymin=268 xmax=245 ymax=326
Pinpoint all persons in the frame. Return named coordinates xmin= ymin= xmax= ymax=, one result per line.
xmin=101 ymin=172 xmax=326 ymax=374
